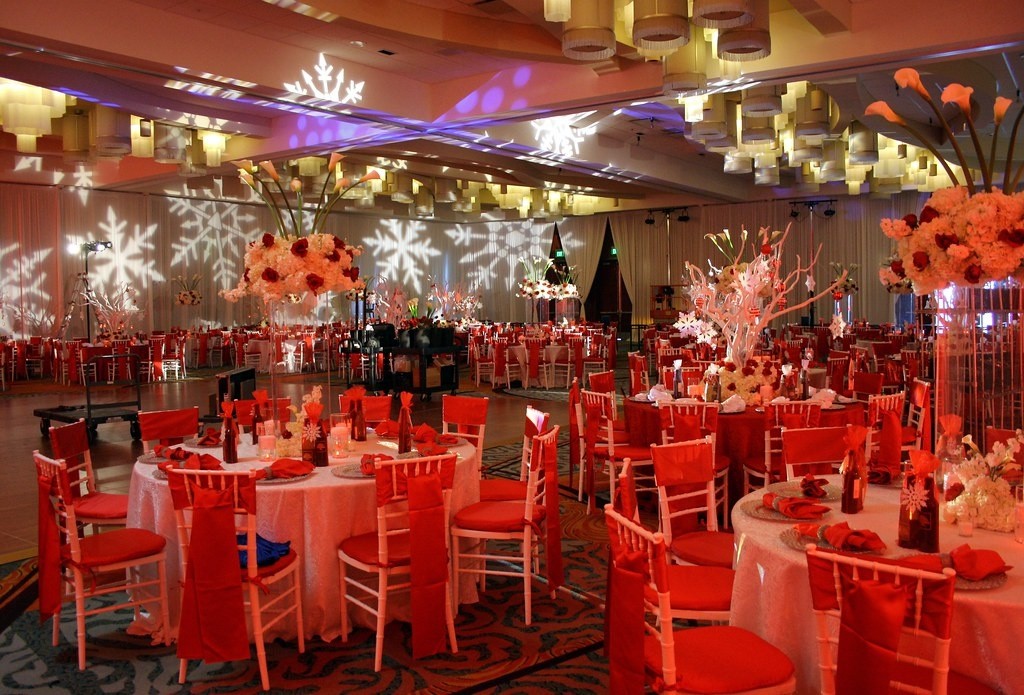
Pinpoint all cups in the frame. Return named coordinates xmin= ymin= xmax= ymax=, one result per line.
xmin=259 ymin=425 xmax=278 ymax=461
xmin=330 ymin=412 xmax=352 ymax=458
xmin=1014 ymin=484 xmax=1024 ymax=544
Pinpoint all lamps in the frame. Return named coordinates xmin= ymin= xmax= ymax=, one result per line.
xmin=336 ymin=161 xmax=599 ymax=222
xmin=289 ymin=156 xmax=327 ymax=177
xmin=196 ymin=129 xmax=231 ymax=168
xmin=0 ymin=76 xmax=77 ymax=153
xmin=678 ymin=92 xmax=708 ymax=122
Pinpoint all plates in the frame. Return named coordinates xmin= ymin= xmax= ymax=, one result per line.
xmin=739 ymin=478 xmax=837 ymax=552
xmin=136 ymin=434 xmax=467 ymax=485
xmin=627 ymin=385 xmax=860 ymax=414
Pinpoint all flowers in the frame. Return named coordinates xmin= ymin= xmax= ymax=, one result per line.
xmin=704 ymin=226 xmax=784 ymax=302
xmin=827 ymin=261 xmax=859 ymax=296
xmin=513 ymin=256 xmax=580 ymax=300
xmin=407 ymin=275 xmax=484 ymax=322
xmin=221 ymin=152 xmax=380 ymax=307
xmin=866 ymin=68 xmax=1024 ymax=297
xmin=171 ymin=273 xmax=204 ymax=305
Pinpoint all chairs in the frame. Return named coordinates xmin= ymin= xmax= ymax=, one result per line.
xmin=33 ymin=387 xmax=561 ymax=689
xmin=468 ymin=319 xmax=1024 ymax=695
xmin=0 ymin=316 xmax=467 ymax=391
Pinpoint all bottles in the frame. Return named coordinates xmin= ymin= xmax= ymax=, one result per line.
xmin=300 ymin=418 xmax=329 ymax=467
xmin=398 ymin=408 xmax=411 ymax=454
xmin=346 ymin=399 xmax=367 ymax=441
xmin=841 ymin=450 xmax=865 ymax=514
xmin=672 ymin=370 xmax=684 ymax=399
xmin=703 ymin=374 xmax=721 ymax=403
xmin=897 ymin=459 xmax=942 ymax=553
xmin=798 ymin=369 xmax=810 ymax=400
xmin=252 ymin=403 xmax=265 ymax=445
xmin=222 ymin=416 xmax=238 ymax=464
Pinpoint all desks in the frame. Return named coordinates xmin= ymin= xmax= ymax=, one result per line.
xmin=82 ymin=340 xmax=154 ymax=383
xmin=729 ymin=472 xmax=1024 ymax=694
xmin=126 ymin=428 xmax=481 ymax=645
xmin=623 ymin=389 xmax=864 ymax=481
